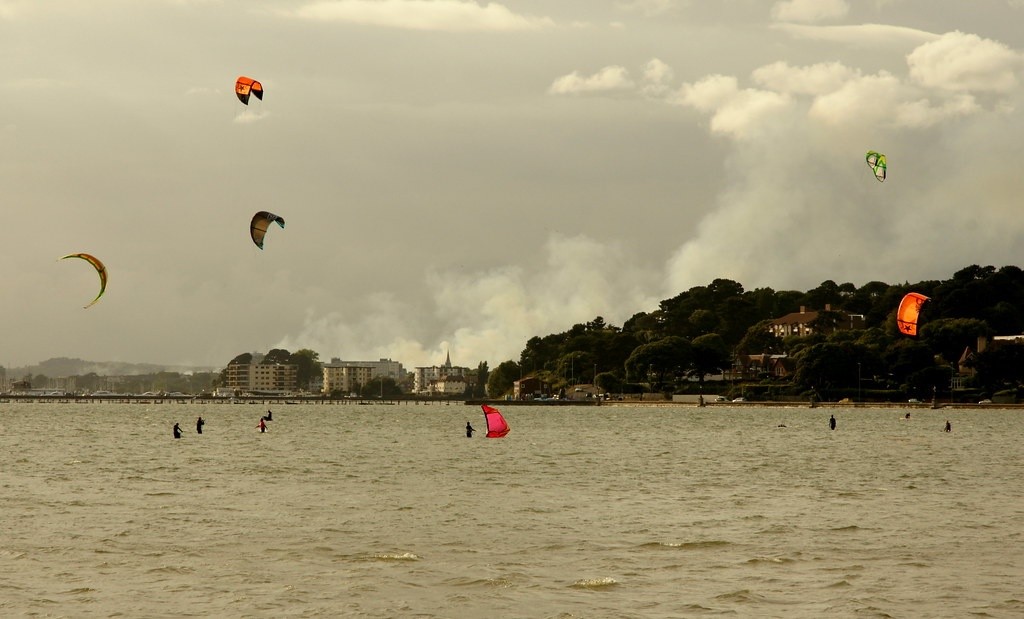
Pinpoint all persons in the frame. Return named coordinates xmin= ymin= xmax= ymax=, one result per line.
xmin=263 ymin=411 xmax=272 ymax=421
xmin=945 ymin=421 xmax=951 ymax=432
xmin=829 ymin=415 xmax=836 ymax=430
xmin=257 ymin=418 xmax=267 ymax=433
xmin=173 ymin=423 xmax=183 ymax=438
xmin=905 ymin=413 xmax=910 ymax=419
xmin=197 ymin=417 xmax=204 ymax=434
xmin=466 ymin=422 xmax=476 ymax=437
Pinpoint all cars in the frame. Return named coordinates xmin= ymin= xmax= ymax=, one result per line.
xmin=533 ymin=387 xmax=612 ymax=402
xmin=979 ymin=398 xmax=993 ymax=404
xmin=715 ymin=396 xmax=729 ymax=403
xmin=908 ymin=398 xmax=919 ymax=404
xmin=48 ymin=388 xmax=254 ymax=398
xmin=733 ymin=396 xmax=747 ymax=404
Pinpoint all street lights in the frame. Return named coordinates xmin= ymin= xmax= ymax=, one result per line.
xmin=650 ymin=364 xmax=652 ymax=392
xmin=857 ymin=363 xmax=861 ymax=402
xmin=732 ymin=364 xmax=736 ymax=399
xmin=594 ymin=364 xmax=597 ymax=387
xmin=950 ymin=362 xmax=954 ymax=403
